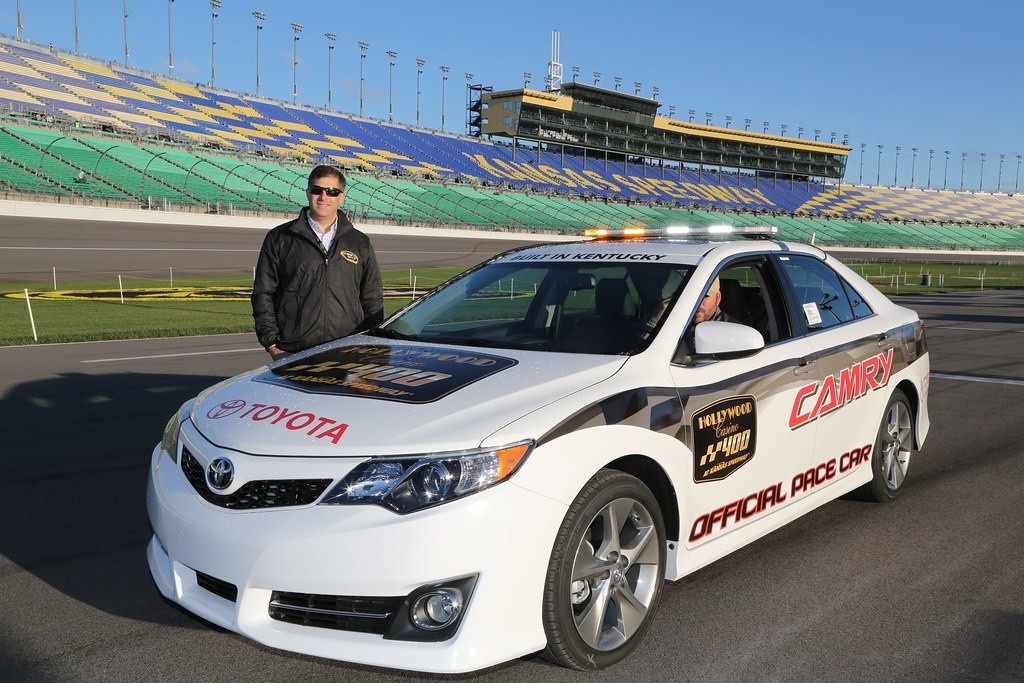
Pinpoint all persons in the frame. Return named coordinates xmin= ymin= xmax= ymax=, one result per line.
xmin=252 ymin=165 xmax=385 ymax=360
xmin=638 ymin=275 xmax=745 ymax=359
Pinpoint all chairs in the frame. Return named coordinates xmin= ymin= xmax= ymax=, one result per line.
xmin=717 ymin=278 xmax=755 ymax=326
xmin=555 ymin=278 xmax=636 ymax=356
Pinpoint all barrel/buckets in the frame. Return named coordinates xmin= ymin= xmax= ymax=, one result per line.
xmin=921 ymin=274 xmax=931 ymax=285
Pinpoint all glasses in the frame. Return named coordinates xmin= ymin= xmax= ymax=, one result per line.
xmin=310 ymin=185 xmax=344 ymax=197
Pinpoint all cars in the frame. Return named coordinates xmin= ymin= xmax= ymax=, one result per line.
xmin=144 ymin=225 xmax=931 ymax=674
